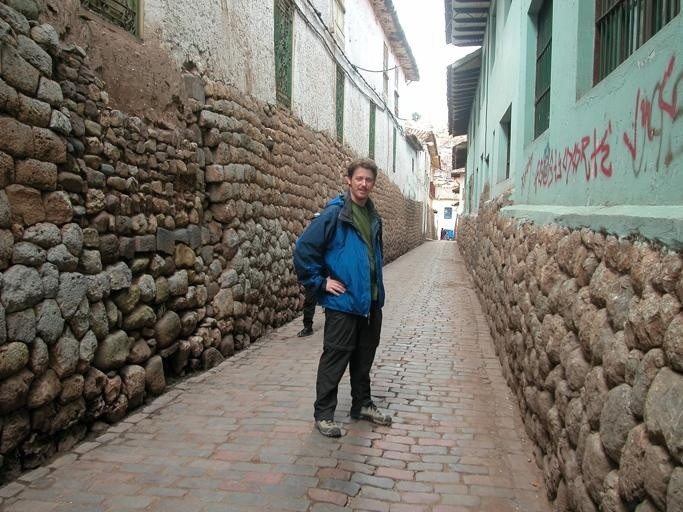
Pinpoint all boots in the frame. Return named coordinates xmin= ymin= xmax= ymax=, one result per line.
xmin=297 ymin=321 xmax=313 ymax=337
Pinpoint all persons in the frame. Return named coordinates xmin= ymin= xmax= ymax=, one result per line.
xmin=298 ymin=211 xmax=321 ymax=338
xmin=294 ymin=159 xmax=392 ymax=436
xmin=440 ymin=227 xmax=443 ymax=240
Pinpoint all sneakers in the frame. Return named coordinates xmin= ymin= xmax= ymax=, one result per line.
xmin=316 ymin=419 xmax=341 ymax=438
xmin=351 ymin=404 xmax=392 ymax=426
